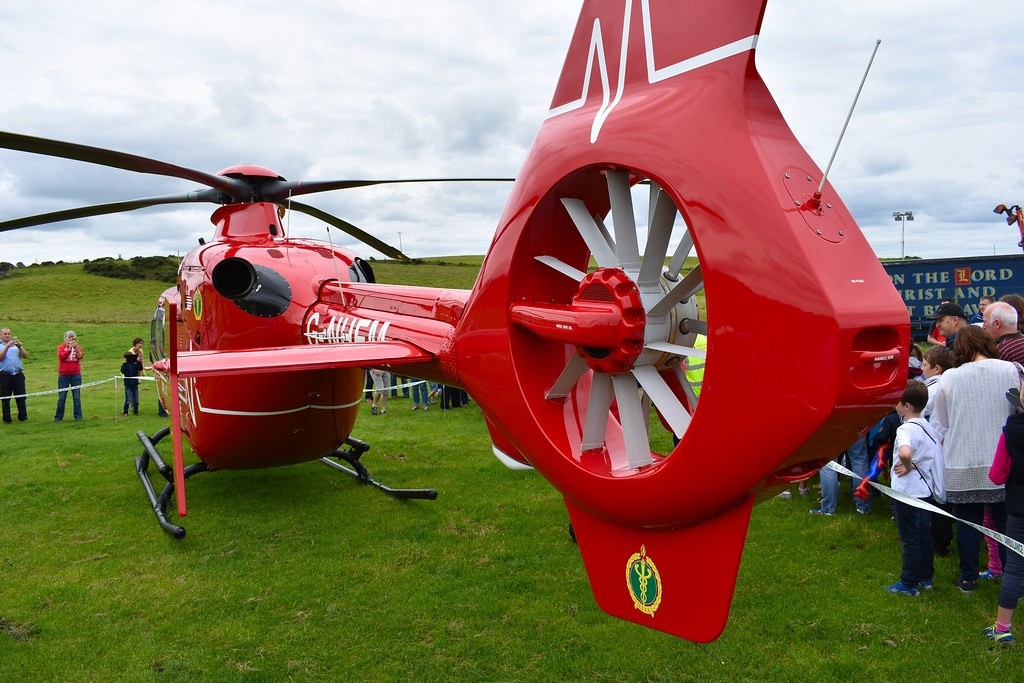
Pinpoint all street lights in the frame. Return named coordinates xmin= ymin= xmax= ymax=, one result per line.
xmin=893 ymin=211 xmax=913 ymax=260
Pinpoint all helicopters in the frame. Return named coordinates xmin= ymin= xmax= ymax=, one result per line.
xmin=0 ymin=1 xmax=911 ymax=645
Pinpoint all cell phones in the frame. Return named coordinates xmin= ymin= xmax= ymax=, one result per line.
xmin=72 ymin=338 xmax=76 ymax=341
xmin=13 ymin=337 xmax=17 ymax=344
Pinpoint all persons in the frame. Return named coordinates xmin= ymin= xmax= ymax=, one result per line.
xmin=883 ymin=378 xmax=945 ymax=596
xmin=123 ymin=338 xmax=146 ymax=414
xmin=0 ymin=326 xmax=27 ymax=423
xmin=365 ymin=367 xmax=468 ymax=415
xmin=120 ymin=351 xmax=143 ymax=416
xmin=54 ymin=331 xmax=84 ymax=421
xmin=928 ymin=326 xmax=1024 ymax=594
xmin=781 ymin=295 xmax=1024 ymax=644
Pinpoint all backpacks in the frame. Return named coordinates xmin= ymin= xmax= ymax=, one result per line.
xmin=907 ymin=422 xmax=946 ymax=504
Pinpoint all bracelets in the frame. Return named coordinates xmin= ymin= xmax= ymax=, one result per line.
xmin=76 ymin=350 xmax=80 ymax=353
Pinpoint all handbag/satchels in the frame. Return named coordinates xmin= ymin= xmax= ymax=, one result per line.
xmin=988 ymin=426 xmax=1011 ymax=484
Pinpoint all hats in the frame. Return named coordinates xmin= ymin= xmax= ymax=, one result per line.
xmin=926 ymin=303 xmax=968 ymax=319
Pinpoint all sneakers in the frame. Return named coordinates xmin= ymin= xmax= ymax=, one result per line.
xmin=883 ymin=578 xmax=920 ymax=598
xmin=979 ymin=569 xmax=1001 ymax=581
xmin=982 ymin=622 xmax=1015 ymax=646
xmin=918 ymin=579 xmax=933 ymax=590
xmin=955 ymin=575 xmax=977 ymax=593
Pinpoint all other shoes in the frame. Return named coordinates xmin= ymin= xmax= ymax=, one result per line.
xmin=371 ymin=404 xmax=377 ymax=415
xmin=380 ymin=410 xmax=385 ymax=414
xmin=123 ymin=411 xmax=128 ymax=416
xmin=365 ymin=399 xmax=372 ymax=403
xmin=422 ymin=406 xmax=428 ymax=410
xmin=809 ymin=507 xmax=836 ymax=517
xmin=412 ymin=406 xmax=420 ymax=410
xmin=936 ymin=543 xmax=950 ymax=558
xmin=778 ymin=492 xmax=793 ymax=499
xmin=857 ymin=509 xmax=871 ymax=514
xmin=798 ymin=486 xmax=809 ymax=496
xmin=133 ymin=412 xmax=141 ymax=416
